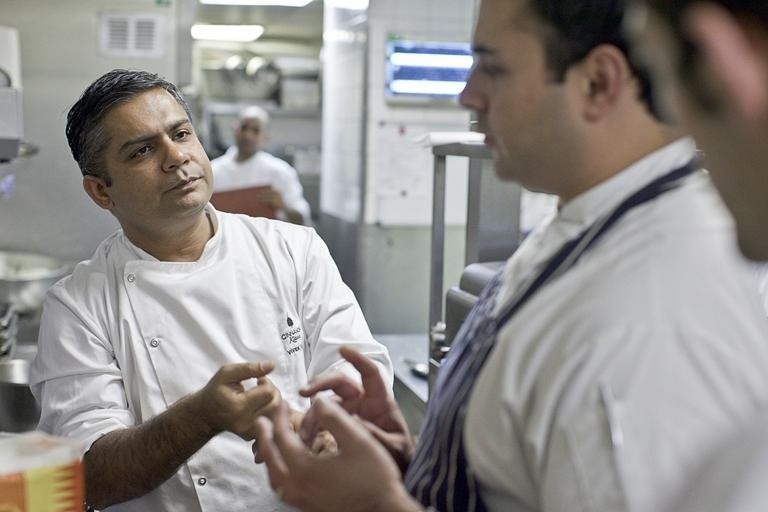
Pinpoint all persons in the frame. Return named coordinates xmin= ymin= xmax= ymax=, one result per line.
xmin=209 ymin=102 xmax=315 ymax=230
xmin=254 ymin=0 xmax=743 ymax=511
xmin=626 ymin=0 xmax=767 ymax=511
xmin=24 ymin=69 xmax=397 ymax=510
